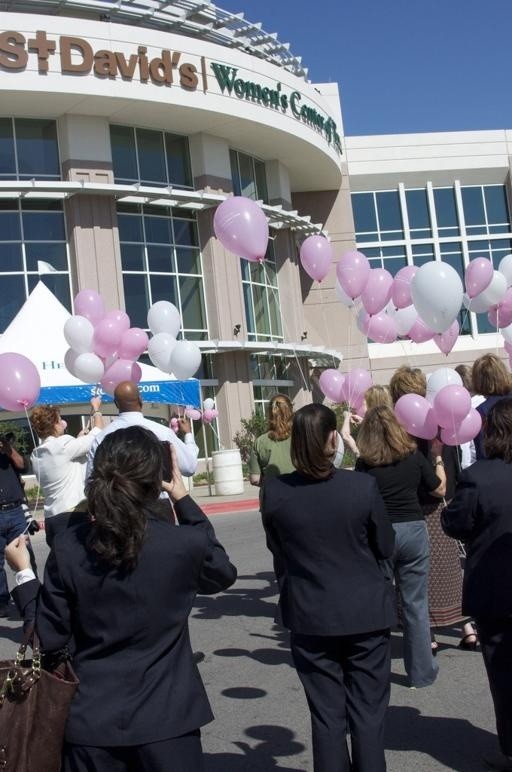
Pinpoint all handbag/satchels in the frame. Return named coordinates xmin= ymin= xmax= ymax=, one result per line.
xmin=1 ymin=618 xmax=80 ymax=772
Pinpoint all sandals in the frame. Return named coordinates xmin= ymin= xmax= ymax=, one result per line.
xmin=458 ymin=632 xmax=478 ymax=650
xmin=432 ymin=648 xmax=437 ymax=656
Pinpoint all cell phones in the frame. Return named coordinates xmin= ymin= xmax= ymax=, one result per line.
xmin=156 ymin=441 xmax=173 ymax=485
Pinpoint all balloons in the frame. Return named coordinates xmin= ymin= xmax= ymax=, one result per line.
xmin=147 ymin=301 xmax=181 ymax=339
xmin=387 ymin=303 xmax=419 ymax=338
xmin=503 ymin=341 xmax=511 ymax=354
xmin=336 ymin=250 xmax=370 ymax=299
xmin=440 ymin=407 xmax=482 ymax=445
xmin=352 ymin=296 xmax=370 ymax=333
xmin=319 ymin=369 xmax=346 ymax=403
xmin=211 ymin=409 xmax=219 ymax=418
xmin=432 ymin=320 xmax=459 ymax=357
xmin=335 ymin=278 xmax=362 ymax=309
xmin=424 ymin=368 xmax=463 ymax=404
xmin=213 ymin=196 xmax=268 ymax=263
xmin=463 ymin=269 xmax=506 ymax=313
xmin=408 ymin=316 xmax=436 ymax=345
xmin=394 ymin=393 xmax=438 ymax=440
xmin=64 ymin=315 xmax=94 ymax=353
xmin=64 ymin=348 xmax=77 ymax=377
xmin=148 ymin=333 xmax=177 ymax=374
xmin=299 ymin=235 xmax=332 ymax=282
xmin=362 ymin=268 xmax=394 ymax=317
xmin=171 ymin=339 xmax=202 ymax=381
xmin=74 ymin=352 xmax=105 ymax=384
xmin=390 ymin=265 xmax=420 ymax=310
xmin=117 ymin=327 xmax=148 ymax=362
xmin=498 ymin=254 xmax=512 ymax=288
xmin=0 ymin=352 xmax=40 ymax=411
xmin=432 ymin=384 xmax=471 ymax=428
xmin=92 ymin=318 xmax=122 ymax=358
xmin=97 ymin=308 xmax=130 ymax=348
xmin=500 ymin=323 xmax=512 ymax=343
xmin=101 ymin=360 xmax=141 ymax=398
xmin=488 ymin=288 xmax=512 ymax=328
xmin=362 ymin=313 xmax=397 ymax=344
xmin=344 ymin=368 xmax=372 ymax=409
xmin=203 ymin=398 xmax=214 ymax=410
xmin=172 ymin=406 xmax=185 ymax=416
xmin=74 ymin=289 xmax=104 ymax=326
xmin=410 ymin=261 xmax=463 ymax=335
xmin=204 ymin=410 xmax=212 ymax=419
xmin=185 ymin=409 xmax=201 ymax=421
xmin=465 ymin=256 xmax=493 ymax=298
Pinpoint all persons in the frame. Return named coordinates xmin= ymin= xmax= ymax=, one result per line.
xmin=471 ymin=352 xmax=510 ymax=461
xmin=260 ymin=403 xmax=396 ymax=771
xmin=354 ymin=405 xmax=447 ymax=688
xmin=84 ymin=380 xmax=199 ymax=525
xmin=440 ymin=398 xmax=512 ymax=772
xmin=454 ymin=363 xmax=489 ymax=470
xmin=30 ymin=396 xmax=105 ymax=549
xmin=250 ymin=395 xmax=298 ymax=512
xmin=5 ymin=425 xmax=237 ymax=771
xmin=389 ymin=365 xmax=477 ymax=655
xmin=342 ymin=384 xmax=388 ymax=449
xmin=1 ymin=434 xmax=38 ymax=618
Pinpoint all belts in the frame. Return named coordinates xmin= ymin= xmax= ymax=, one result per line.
xmin=0 ymin=501 xmax=26 ymax=509
